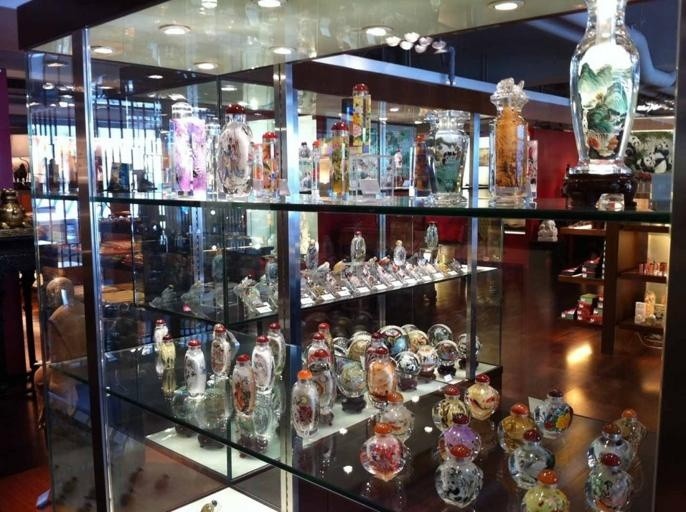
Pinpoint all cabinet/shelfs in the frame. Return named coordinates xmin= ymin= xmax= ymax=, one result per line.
xmin=14 ymin=2 xmax=684 ymax=512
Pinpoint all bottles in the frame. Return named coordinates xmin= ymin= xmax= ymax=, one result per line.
xmin=152 ymin=319 xmax=338 ymax=441
xmin=358 ymin=373 xmax=647 ymax=511
xmin=426 ymin=221 xmax=439 ymax=248
xmin=332 ymin=120 xmax=351 ymax=193
xmin=490 ymin=76 xmax=529 ymax=196
xmin=211 ymin=239 xmax=318 ymax=281
xmin=394 ymin=239 xmax=406 ymax=266
xmin=252 ymin=129 xmax=319 ymax=196
xmin=350 ymin=230 xmax=367 ymax=262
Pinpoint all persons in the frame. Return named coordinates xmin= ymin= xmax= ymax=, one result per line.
xmin=33 ymin=276 xmax=87 ymax=429
